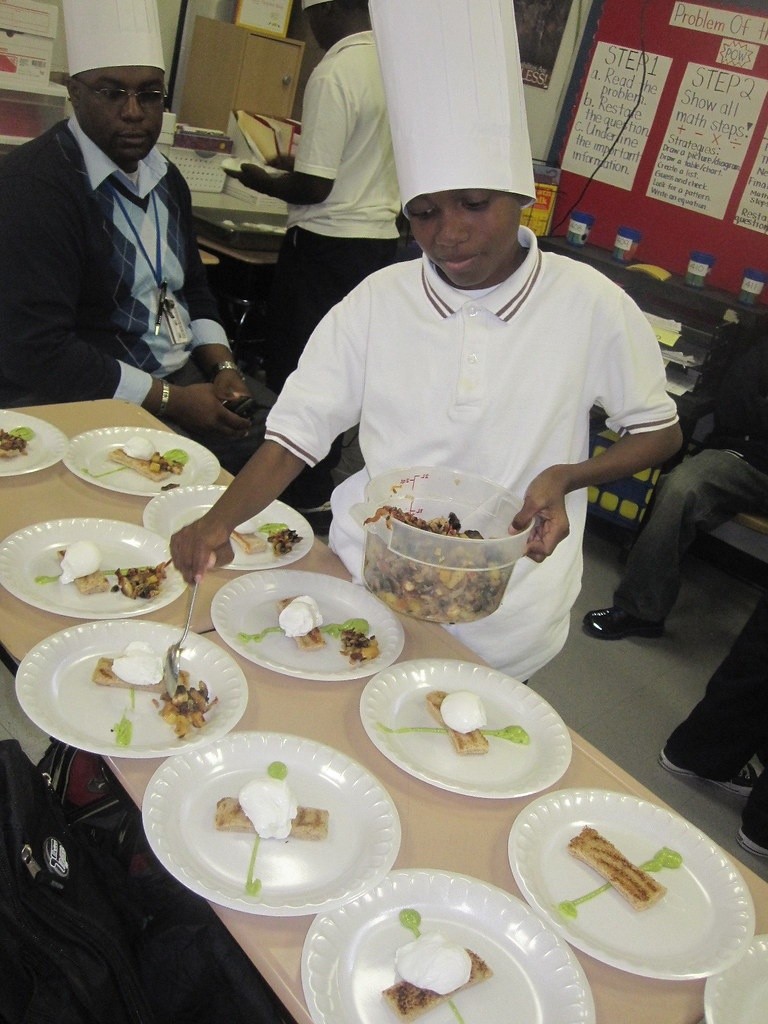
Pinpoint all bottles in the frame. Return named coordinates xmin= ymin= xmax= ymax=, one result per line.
xmin=684 ymin=251 xmax=715 ymax=290
xmin=736 ymin=268 xmax=768 ymax=307
xmin=566 ymin=210 xmax=595 ymax=247
xmin=612 ymin=224 xmax=641 ymax=264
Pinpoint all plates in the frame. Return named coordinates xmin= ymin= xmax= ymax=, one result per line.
xmin=211 ymin=569 xmax=406 ymax=680
xmin=704 ymin=934 xmax=768 ymax=1024
xmin=507 ymin=788 xmax=756 ymax=981
xmin=0 ymin=409 xmax=69 ymax=477
xmin=141 ymin=730 xmax=401 ymax=916
xmin=220 ymin=163 xmax=290 ymax=180
xmin=0 ymin=517 xmax=190 ymax=619
xmin=143 ymin=485 xmax=313 ymax=571
xmin=301 ymin=869 xmax=596 ymax=1024
xmin=360 ymin=658 xmax=574 ymax=797
xmin=16 ymin=619 xmax=249 ymax=758
xmin=61 ymin=426 xmax=221 ymax=498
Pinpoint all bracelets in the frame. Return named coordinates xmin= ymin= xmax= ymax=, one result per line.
xmin=208 ymin=360 xmax=246 ymax=383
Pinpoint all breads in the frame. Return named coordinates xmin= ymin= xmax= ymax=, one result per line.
xmin=108 ymin=448 xmax=171 ymax=482
xmin=380 ymin=947 xmax=494 ymax=1023
xmin=215 ymin=796 xmax=329 ymax=841
xmin=0 ymin=446 xmax=21 ymax=456
xmin=58 ymin=549 xmax=110 ymax=593
xmin=571 ymin=826 xmax=668 ymax=911
xmin=230 ymin=530 xmax=266 ymax=554
xmin=93 ymin=656 xmax=190 ymax=694
xmin=278 ymin=596 xmax=324 ymax=648
xmin=425 ymin=690 xmax=490 ymax=757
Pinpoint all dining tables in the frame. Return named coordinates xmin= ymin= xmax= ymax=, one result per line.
xmin=1 ymin=399 xmax=768 ymax=1024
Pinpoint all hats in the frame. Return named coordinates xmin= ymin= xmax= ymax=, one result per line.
xmin=369 ymin=0 xmax=537 ymax=219
xmin=64 ymin=0 xmax=166 ymax=77
xmin=300 ymin=0 xmax=333 ymax=11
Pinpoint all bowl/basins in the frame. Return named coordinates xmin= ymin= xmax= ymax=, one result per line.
xmin=349 ymin=466 xmax=535 ymax=624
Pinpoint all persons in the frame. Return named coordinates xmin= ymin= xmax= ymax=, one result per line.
xmin=584 ymin=309 xmax=768 ymax=640
xmin=224 ymin=2 xmax=411 ymax=534
xmin=0 ymin=0 xmax=306 ymax=505
xmin=660 ymin=599 xmax=768 ymax=856
xmin=172 ymin=1 xmax=684 ymax=688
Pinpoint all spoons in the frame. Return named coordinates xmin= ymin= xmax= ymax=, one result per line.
xmin=164 ymin=578 xmax=199 ymax=698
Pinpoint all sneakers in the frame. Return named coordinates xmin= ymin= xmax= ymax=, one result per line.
xmin=735 ymin=810 xmax=768 ymax=857
xmin=661 ymin=734 xmax=763 ymax=795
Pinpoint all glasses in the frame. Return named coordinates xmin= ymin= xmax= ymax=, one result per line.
xmin=72 ymin=75 xmax=168 ymax=110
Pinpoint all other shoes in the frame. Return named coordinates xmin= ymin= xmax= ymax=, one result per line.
xmin=278 ymin=468 xmax=335 ymax=514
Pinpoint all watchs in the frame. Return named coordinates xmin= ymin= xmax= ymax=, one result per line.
xmin=158 ymin=376 xmax=170 ymax=412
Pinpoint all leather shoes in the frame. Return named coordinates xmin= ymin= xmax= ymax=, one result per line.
xmin=583 ymin=607 xmax=655 ymax=640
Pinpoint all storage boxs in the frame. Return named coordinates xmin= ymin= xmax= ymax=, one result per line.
xmin=166 ymin=146 xmax=229 ymax=193
xmin=586 ymin=417 xmax=662 ymax=531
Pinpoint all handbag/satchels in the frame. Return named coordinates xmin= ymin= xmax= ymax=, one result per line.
xmin=0 ymin=736 xmax=294 ymax=1024
